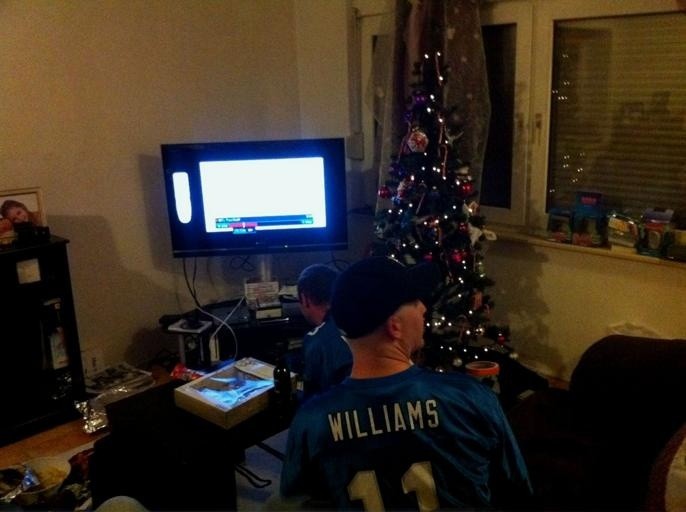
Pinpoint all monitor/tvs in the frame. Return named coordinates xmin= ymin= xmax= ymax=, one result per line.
xmin=160 ymin=136 xmax=348 ymax=258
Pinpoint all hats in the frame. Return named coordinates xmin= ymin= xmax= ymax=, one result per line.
xmin=329 ymin=256 xmax=442 ymax=339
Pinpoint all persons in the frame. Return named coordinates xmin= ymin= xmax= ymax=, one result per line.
xmin=279 ymin=257 xmax=541 ymax=512
xmin=0 ymin=199 xmax=40 ymax=226
xmin=293 ymin=263 xmax=352 ymax=397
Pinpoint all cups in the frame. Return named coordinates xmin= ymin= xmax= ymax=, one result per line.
xmin=14 ymin=457 xmax=71 ymax=505
xmin=464 ymin=360 xmax=501 ymax=392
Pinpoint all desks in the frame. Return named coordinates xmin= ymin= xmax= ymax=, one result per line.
xmin=106 ymin=378 xmax=303 ymax=512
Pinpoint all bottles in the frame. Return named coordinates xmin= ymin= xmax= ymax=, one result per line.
xmin=271 ymin=357 xmax=291 ymax=409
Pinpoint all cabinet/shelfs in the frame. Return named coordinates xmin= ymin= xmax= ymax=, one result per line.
xmin=0 ymin=234 xmax=85 ymax=448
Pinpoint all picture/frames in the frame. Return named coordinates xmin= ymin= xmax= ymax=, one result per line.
xmin=0 ymin=187 xmax=46 ymax=226
